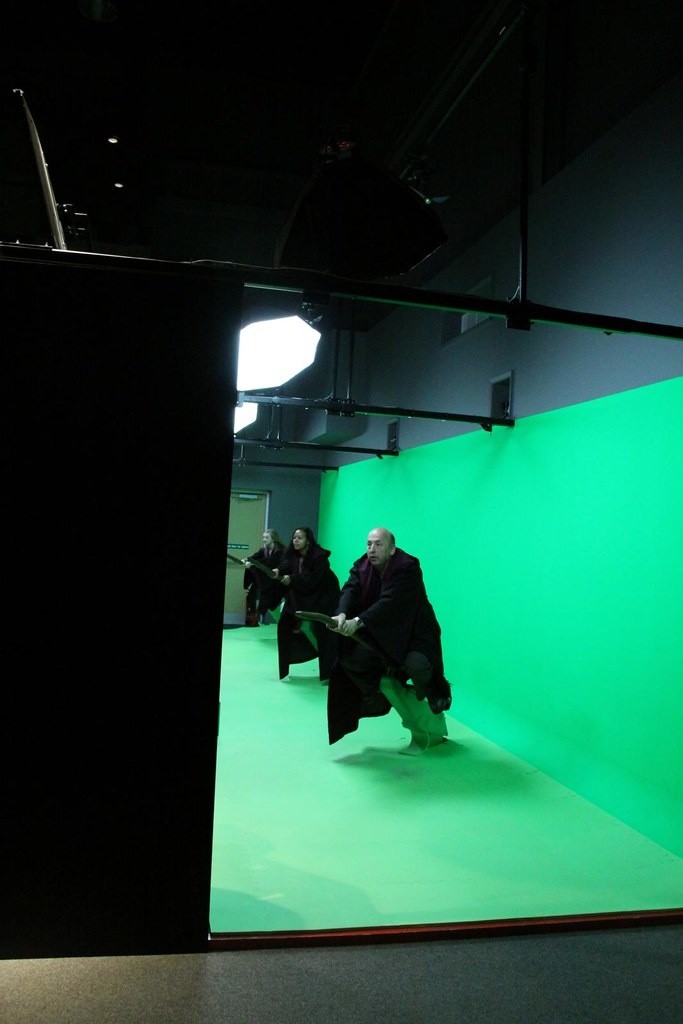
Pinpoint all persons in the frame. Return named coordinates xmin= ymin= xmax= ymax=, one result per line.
xmin=325 ymin=527 xmax=453 ymax=746
xmin=241 ymin=528 xmax=287 ymax=628
xmin=271 ymin=527 xmax=341 ymax=680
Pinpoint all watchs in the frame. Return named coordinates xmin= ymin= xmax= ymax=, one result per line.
xmin=355 ymin=616 xmax=363 ymax=626
